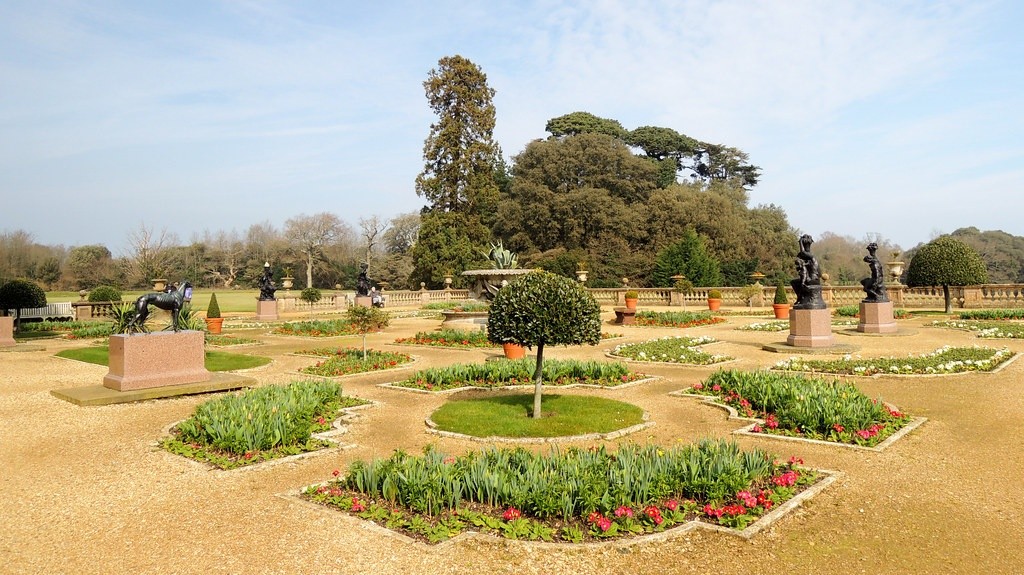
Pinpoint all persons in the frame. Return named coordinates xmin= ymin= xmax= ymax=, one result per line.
xmin=790 ymin=237 xmax=826 ymax=305
xmin=862 ymin=255 xmax=883 ymax=301
xmin=368 ymin=287 xmax=381 ymax=308
xmin=256 ymin=262 xmax=276 ymax=300
xmin=357 ymin=263 xmax=370 ymax=295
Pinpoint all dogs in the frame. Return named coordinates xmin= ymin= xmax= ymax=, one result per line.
xmin=127 ymin=279 xmax=193 ymax=335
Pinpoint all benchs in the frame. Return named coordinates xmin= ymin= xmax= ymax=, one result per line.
xmin=6 ymin=302 xmax=77 ymax=323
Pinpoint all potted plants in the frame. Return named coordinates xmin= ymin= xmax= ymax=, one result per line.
xmin=772 ymin=281 xmax=790 ymax=319
xmin=706 ymin=290 xmax=723 ymax=311
xmin=203 ymin=292 xmax=224 ymax=336
xmin=575 ymin=262 xmax=588 ymax=282
xmin=833 ymin=305 xmax=912 ymax=320
xmin=625 ymin=291 xmax=639 ymax=309
xmin=960 ymin=308 xmax=1024 ymax=322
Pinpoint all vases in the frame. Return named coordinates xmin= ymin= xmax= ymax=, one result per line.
xmin=502 ymin=342 xmax=526 ymax=360
